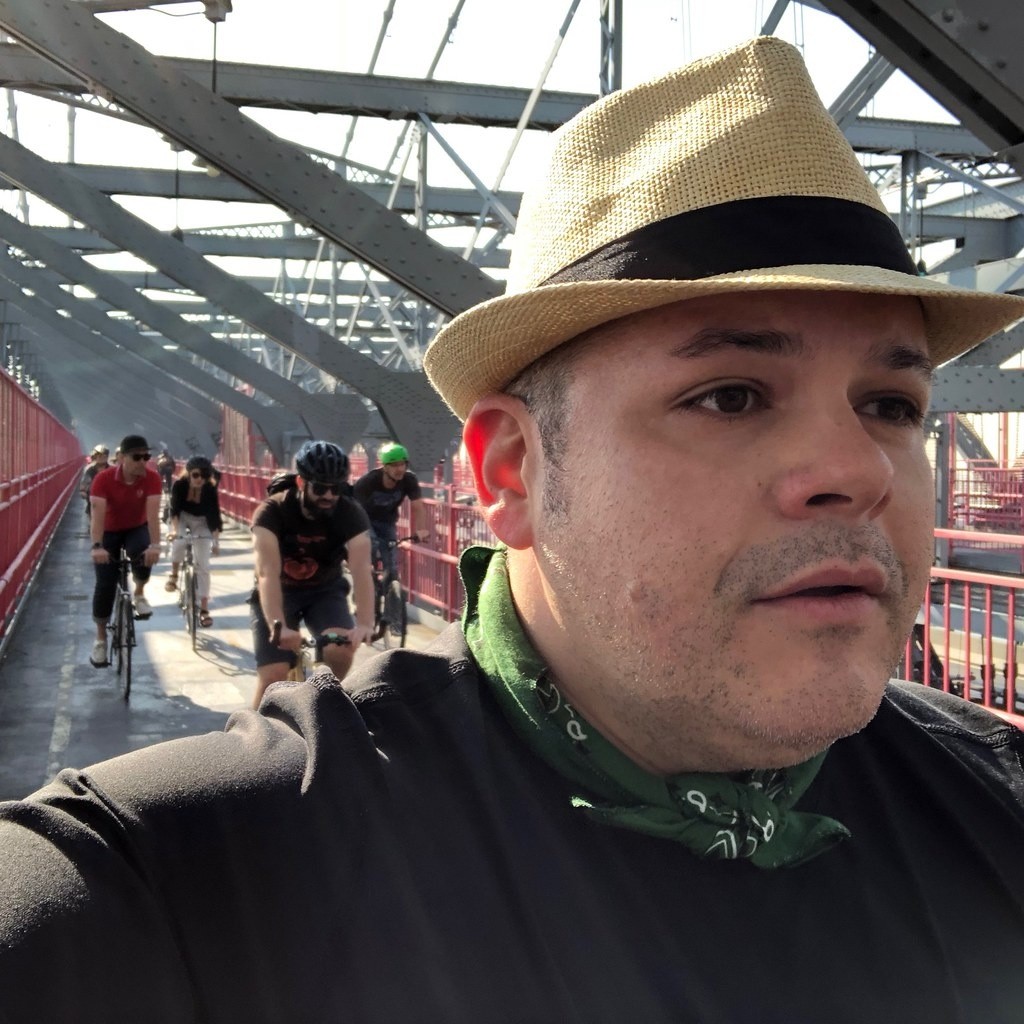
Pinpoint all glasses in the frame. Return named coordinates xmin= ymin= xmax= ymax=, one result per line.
xmin=308 ymin=481 xmax=346 ymax=497
xmin=127 ymin=451 xmax=152 ymax=462
xmin=190 ymin=472 xmax=209 ymax=479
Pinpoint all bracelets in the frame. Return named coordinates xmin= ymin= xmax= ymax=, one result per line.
xmin=92 ymin=542 xmax=103 ymax=549
xmin=80 ymin=490 xmax=85 ymax=492
xmin=149 ymin=543 xmax=161 ymax=549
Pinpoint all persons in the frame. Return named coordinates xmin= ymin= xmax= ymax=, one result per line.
xmin=79 ymin=444 xmax=121 ymax=518
xmin=251 ymin=440 xmax=374 ymax=711
xmin=165 ymin=457 xmax=223 ymax=627
xmin=157 ymin=449 xmax=175 ymax=493
xmin=89 ymin=435 xmax=162 ymax=669
xmin=0 ymin=38 xmax=1024 ymax=1024
xmin=350 ymin=443 xmax=430 ymax=635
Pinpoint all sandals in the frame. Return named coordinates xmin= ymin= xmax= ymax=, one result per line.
xmin=197 ymin=608 xmax=214 ymax=627
xmin=165 ymin=573 xmax=179 ymax=592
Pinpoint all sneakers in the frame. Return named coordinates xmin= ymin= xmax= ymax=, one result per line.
xmin=133 ymin=591 xmax=151 ymax=615
xmin=89 ymin=639 xmax=108 ymax=667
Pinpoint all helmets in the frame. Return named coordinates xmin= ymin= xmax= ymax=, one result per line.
xmin=379 ymin=441 xmax=409 ymax=465
xmin=295 ymin=441 xmax=352 ymax=481
xmin=92 ymin=445 xmax=110 ymax=457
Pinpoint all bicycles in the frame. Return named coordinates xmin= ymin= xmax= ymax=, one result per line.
xmin=268 ymin=609 xmax=392 ymax=675
xmin=94 ymin=543 xmax=157 ymax=701
xmin=347 ymin=532 xmax=433 ymax=651
xmin=165 ymin=533 xmax=220 ymax=648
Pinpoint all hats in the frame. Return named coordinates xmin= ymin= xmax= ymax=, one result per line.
xmin=186 ymin=456 xmax=212 ymax=472
xmin=423 ymin=37 xmax=1023 ymax=423
xmin=120 ymin=435 xmax=153 ymax=454
